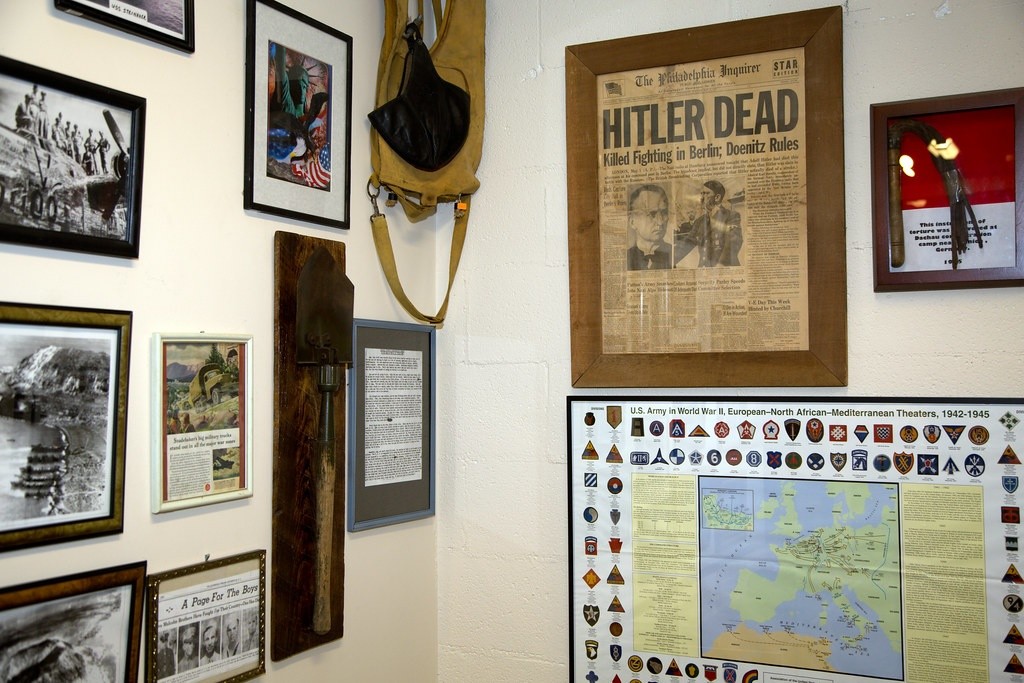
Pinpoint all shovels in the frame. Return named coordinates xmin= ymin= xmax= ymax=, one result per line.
xmin=294 ymin=246 xmax=355 ymax=635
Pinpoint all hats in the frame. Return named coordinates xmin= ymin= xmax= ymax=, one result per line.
xmin=368 ymin=24 xmax=471 ymax=171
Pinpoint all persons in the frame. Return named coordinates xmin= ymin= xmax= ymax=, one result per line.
xmin=674 ymin=180 xmax=743 ymax=267
xmin=14 ymin=84 xmax=111 ymax=175
xmin=627 ymin=184 xmax=672 ymax=271
xmin=157 ymin=611 xmax=260 ymax=680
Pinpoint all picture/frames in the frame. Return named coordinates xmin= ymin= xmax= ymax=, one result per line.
xmin=0 ymin=56 xmax=147 ymax=258
xmin=54 ymin=0 xmax=195 ymax=53
xmin=150 ymin=332 xmax=253 ymax=515
xmin=0 ymin=560 xmax=147 ymax=683
xmin=870 ymin=88 xmax=1024 ymax=292
xmin=243 ymin=0 xmax=353 ymax=229
xmin=0 ymin=301 xmax=133 ymax=555
xmin=145 ymin=549 xmax=267 ymax=683
xmin=566 ymin=6 xmax=850 ymax=388
xmin=346 ymin=319 xmax=436 ymax=533
